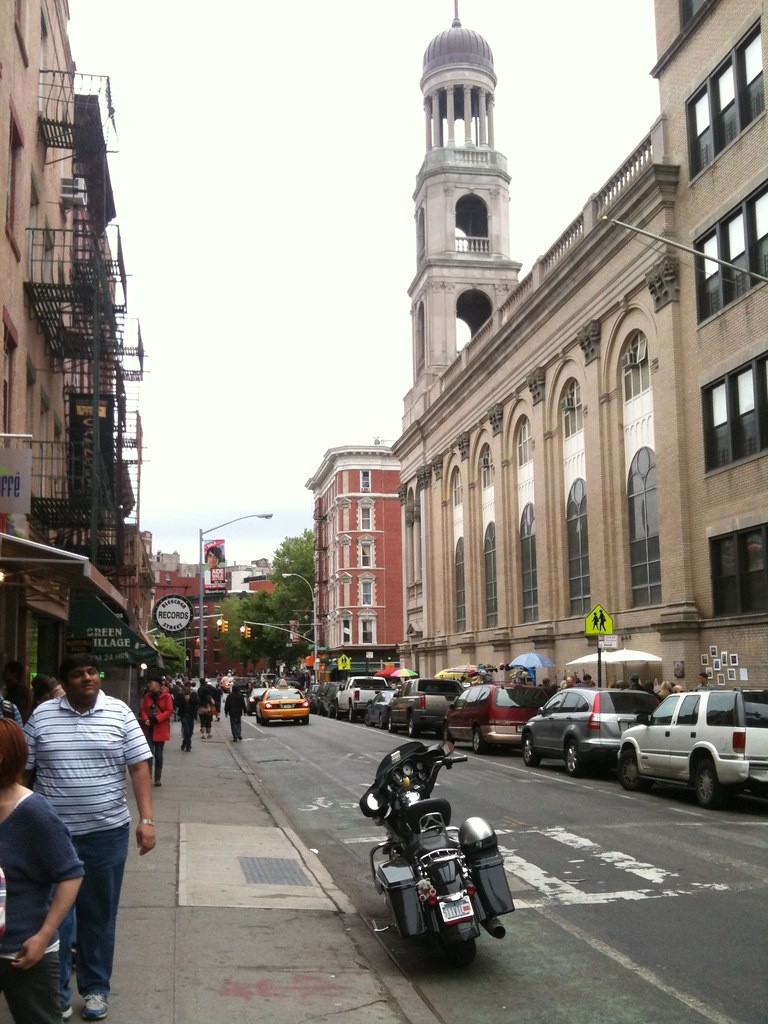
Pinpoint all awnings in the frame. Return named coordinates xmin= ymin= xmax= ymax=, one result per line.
xmin=0 ymin=532 xmax=128 ymax=614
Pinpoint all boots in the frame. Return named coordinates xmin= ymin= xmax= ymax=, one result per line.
xmin=207 ymin=727 xmax=213 ymax=737
xmin=200 ymin=727 xmax=206 ymax=738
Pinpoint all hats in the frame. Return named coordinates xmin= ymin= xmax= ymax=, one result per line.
xmin=147 ymin=673 xmax=162 ymax=683
xmin=631 ymin=673 xmax=640 ymax=680
xmin=468 ymin=663 xmax=512 ymax=684
xmin=31 ymin=675 xmax=49 ymax=692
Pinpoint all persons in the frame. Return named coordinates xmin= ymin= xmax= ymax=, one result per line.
xmin=696 ymin=672 xmax=716 ymax=691
xmin=0 ymin=653 xmax=155 ymax=1024
xmin=203 ymin=546 xmax=223 ymax=585
xmin=225 ymin=684 xmax=247 ymax=742
xmin=388 ymin=679 xmax=402 ymax=689
xmin=246 ymin=680 xmax=266 ymax=698
xmin=160 ymin=672 xmax=223 ymax=752
xmin=140 ymin=678 xmax=173 ymax=786
xmin=609 ymin=674 xmax=683 ymax=703
xmin=221 ymin=668 xmax=233 ymax=677
xmin=462 ymin=674 xmax=480 ymax=689
xmin=289 ymin=670 xmax=315 ymax=696
xmin=536 ymin=674 xmax=595 ymax=698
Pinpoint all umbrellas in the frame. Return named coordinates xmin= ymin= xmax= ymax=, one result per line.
xmin=509 ymin=652 xmax=553 ymax=668
xmin=434 ymin=665 xmax=480 ymax=681
xmin=565 ymin=648 xmax=662 ymax=688
xmin=375 ymin=666 xmax=418 ymax=677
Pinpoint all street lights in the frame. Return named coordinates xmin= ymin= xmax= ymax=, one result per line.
xmin=282 ymin=574 xmax=318 ymax=683
xmin=199 ymin=513 xmax=273 ymax=678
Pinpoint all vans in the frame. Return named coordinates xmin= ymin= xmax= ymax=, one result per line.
xmin=260 ymin=673 xmax=276 ymax=685
xmin=443 ymin=683 xmax=548 ymax=753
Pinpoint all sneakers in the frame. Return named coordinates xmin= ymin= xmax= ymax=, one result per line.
xmin=82 ymin=993 xmax=108 ymax=1020
xmin=62 ymin=1004 xmax=72 ymax=1019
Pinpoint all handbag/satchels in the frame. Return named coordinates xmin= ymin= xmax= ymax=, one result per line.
xmin=209 ymin=705 xmax=218 ymax=715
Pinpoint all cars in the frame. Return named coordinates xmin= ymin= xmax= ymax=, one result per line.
xmin=365 ymin=691 xmax=397 ymax=729
xmin=286 ymin=681 xmax=302 ymax=690
xmin=246 ymin=688 xmax=271 ymax=715
xmin=304 ymin=682 xmax=345 ymax=718
xmin=220 ymin=676 xmax=252 ymax=694
xmin=255 ymin=678 xmax=310 ymax=725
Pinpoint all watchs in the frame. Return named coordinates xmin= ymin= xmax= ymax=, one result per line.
xmin=139 ymin=818 xmax=155 ymax=827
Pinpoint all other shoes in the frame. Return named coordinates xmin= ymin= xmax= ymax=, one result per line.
xmin=238 ymin=734 xmax=242 ymax=740
xmin=233 ymin=735 xmax=237 ymax=742
xmin=187 ymin=747 xmax=190 ymax=752
xmin=181 ymin=739 xmax=186 ymax=750
xmin=155 ymin=777 xmax=161 ymax=786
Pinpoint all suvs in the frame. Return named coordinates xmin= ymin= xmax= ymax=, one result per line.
xmin=616 ymin=688 xmax=768 ymax=808
xmin=387 ymin=678 xmax=467 ymax=738
xmin=520 ymin=687 xmax=661 ymax=776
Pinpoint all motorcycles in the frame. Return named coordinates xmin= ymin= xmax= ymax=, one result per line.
xmin=358 ymin=739 xmax=518 ymax=977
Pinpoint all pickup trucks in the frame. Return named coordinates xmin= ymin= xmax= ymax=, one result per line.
xmin=334 ymin=676 xmax=398 ymax=723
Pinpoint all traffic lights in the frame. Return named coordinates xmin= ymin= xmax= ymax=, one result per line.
xmin=241 ymin=626 xmax=251 ymax=638
xmin=320 ymin=663 xmax=325 ymax=670
xmin=218 ymin=619 xmax=228 ymax=633
xmin=197 ymin=639 xmax=199 ymax=645
